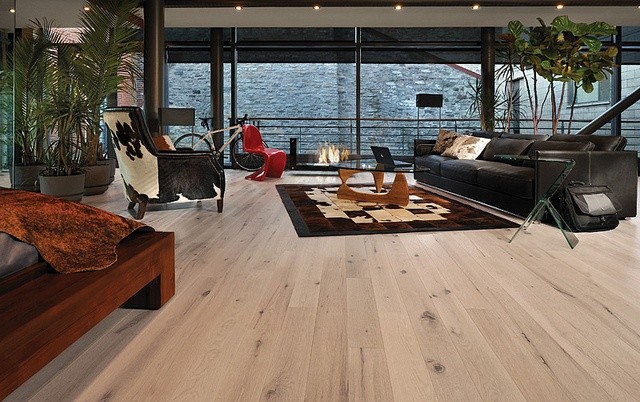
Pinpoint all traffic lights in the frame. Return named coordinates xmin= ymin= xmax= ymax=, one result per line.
xmin=432 ymin=127 xmax=461 ymax=153
xmin=150 ymin=134 xmax=176 ymax=152
xmin=441 ymin=134 xmax=492 ymax=161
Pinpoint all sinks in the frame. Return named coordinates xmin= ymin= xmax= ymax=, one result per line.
xmin=412 ymin=135 xmax=635 ymax=228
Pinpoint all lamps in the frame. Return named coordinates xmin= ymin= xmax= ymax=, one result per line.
xmin=102 ymin=107 xmax=225 ymax=220
xmin=243 ymin=123 xmax=287 ymax=181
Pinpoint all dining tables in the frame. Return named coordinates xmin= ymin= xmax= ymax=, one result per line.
xmin=1 ymin=15 xmax=56 ymax=193
xmin=75 ymin=0 xmax=110 ymax=196
xmin=76 ymin=1 xmax=146 ymax=183
xmin=39 ymin=19 xmax=87 ymax=201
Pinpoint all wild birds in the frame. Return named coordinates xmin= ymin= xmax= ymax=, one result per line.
xmin=370 ymin=146 xmax=413 ymax=167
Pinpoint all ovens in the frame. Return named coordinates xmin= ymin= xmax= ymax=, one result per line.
xmin=1 ymin=186 xmax=175 ymax=402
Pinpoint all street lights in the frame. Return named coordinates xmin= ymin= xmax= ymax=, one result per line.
xmin=557 ymin=181 xmax=622 ymax=231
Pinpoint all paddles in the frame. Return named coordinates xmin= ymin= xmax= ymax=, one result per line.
xmin=274 ymin=183 xmax=521 ymax=238
